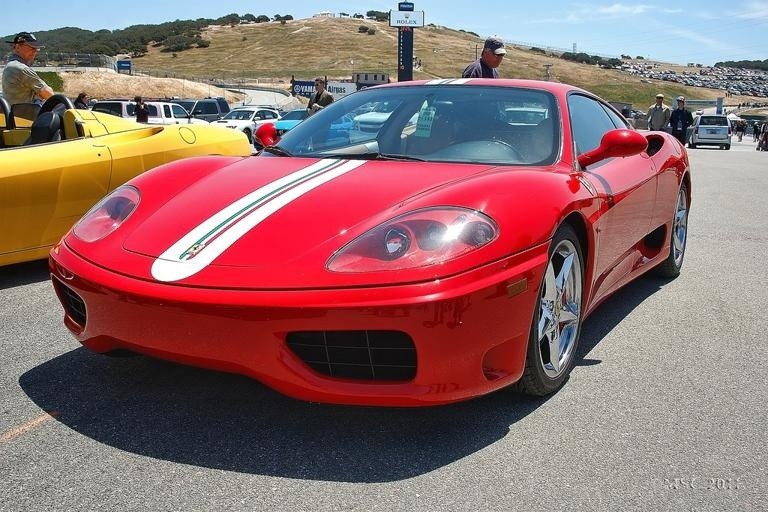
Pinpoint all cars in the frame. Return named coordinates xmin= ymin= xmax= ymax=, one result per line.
xmin=596 ymin=60 xmax=768 ymax=98
xmin=628 ymin=107 xmax=647 ymax=118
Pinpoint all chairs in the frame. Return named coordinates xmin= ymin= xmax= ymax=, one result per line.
xmin=23 ymin=109 xmax=63 ymax=146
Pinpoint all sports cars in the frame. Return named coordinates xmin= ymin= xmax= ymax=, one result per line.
xmin=0 ymin=93 xmax=253 ymax=266
xmin=47 ymin=77 xmax=694 ymax=407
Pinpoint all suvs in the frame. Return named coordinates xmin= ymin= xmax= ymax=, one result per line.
xmin=690 ymin=114 xmax=734 ymax=149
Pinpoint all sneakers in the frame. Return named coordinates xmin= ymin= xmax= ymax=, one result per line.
xmin=736 ymin=137 xmax=768 ymax=151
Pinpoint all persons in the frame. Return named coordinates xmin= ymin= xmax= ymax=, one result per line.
xmin=133 ymin=96 xmax=150 ymax=123
xmin=462 ymin=36 xmax=507 ymax=78
xmin=642 ymin=93 xmax=671 ymax=131
xmin=731 ymin=120 xmax=768 ymax=151
xmin=669 ymin=96 xmax=693 ymax=146
xmin=74 ymin=93 xmax=89 ymax=111
xmin=2 ymin=31 xmax=54 ymax=109
xmin=528 ymin=119 xmax=555 ymax=162
xmin=307 ymin=77 xmax=334 ymax=116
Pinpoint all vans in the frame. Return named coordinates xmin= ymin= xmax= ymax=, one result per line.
xmin=695 ymin=108 xmax=704 ymax=115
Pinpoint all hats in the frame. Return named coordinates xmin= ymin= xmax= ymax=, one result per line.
xmin=4 ymin=32 xmax=46 ymax=49
xmin=676 ymin=96 xmax=685 ymax=102
xmin=656 ymin=93 xmax=665 ymax=99
xmin=483 ymin=34 xmax=508 ymax=58
